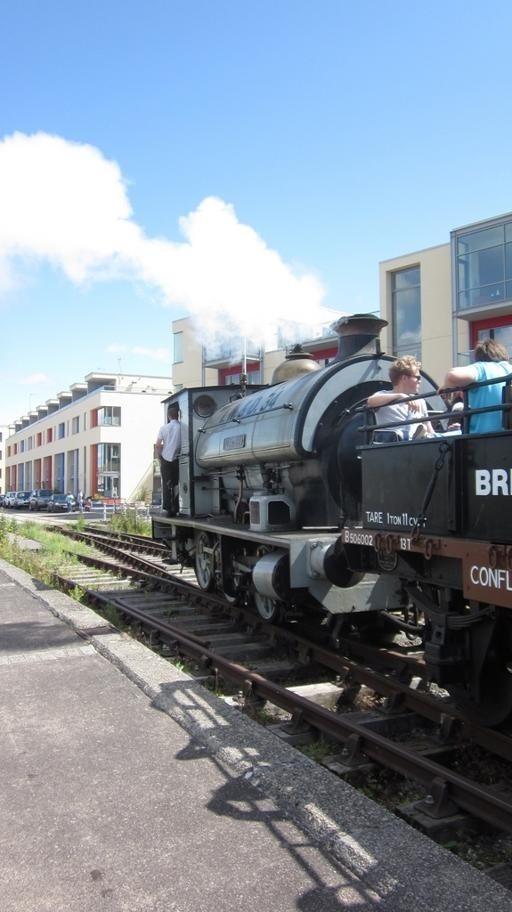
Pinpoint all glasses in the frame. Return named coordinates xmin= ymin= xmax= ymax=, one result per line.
xmin=406 ymin=373 xmax=421 ymax=380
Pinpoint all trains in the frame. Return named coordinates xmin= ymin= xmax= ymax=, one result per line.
xmin=147 ymin=312 xmax=512 ymax=726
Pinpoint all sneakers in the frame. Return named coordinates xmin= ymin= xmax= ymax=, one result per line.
xmin=412 ymin=424 xmax=428 ymax=440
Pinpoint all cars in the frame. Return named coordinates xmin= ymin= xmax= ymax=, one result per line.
xmin=0 ymin=490 xmax=94 ymax=513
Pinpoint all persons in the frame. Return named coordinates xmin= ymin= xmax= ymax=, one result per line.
xmin=156 ymin=407 xmax=181 ymax=517
xmin=446 ymin=391 xmax=465 ymax=432
xmin=436 ymin=337 xmax=512 ymax=436
xmin=64 ymin=486 xmax=118 ymax=514
xmin=366 ymin=355 xmax=436 ymax=441
